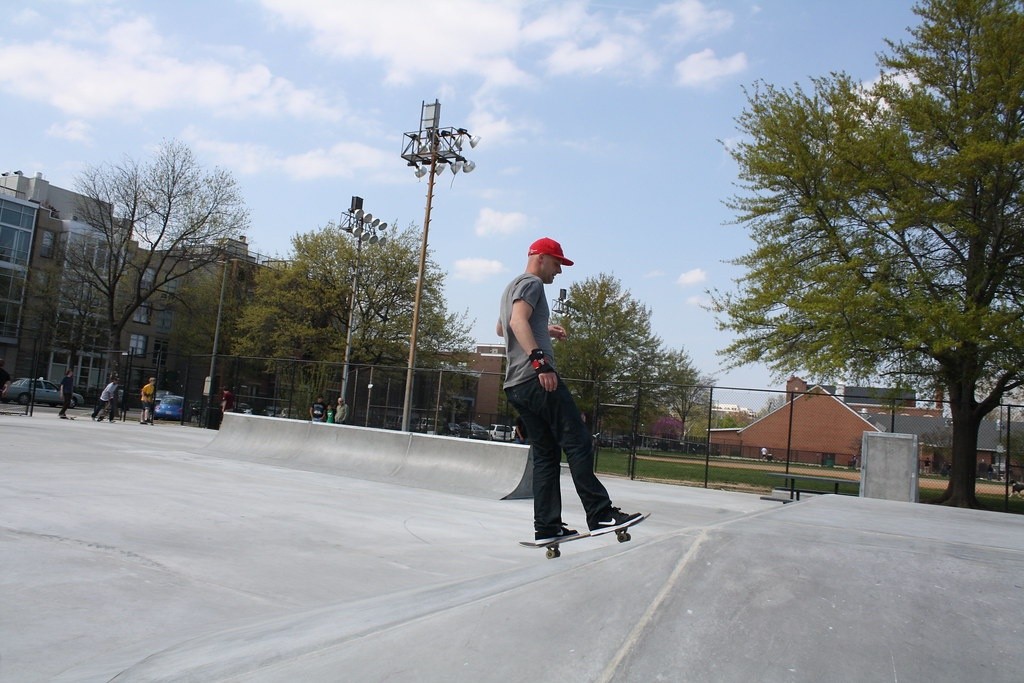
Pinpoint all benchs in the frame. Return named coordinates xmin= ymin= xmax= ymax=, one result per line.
xmin=776 ymin=489 xmax=856 ymax=500
xmin=760 ymin=495 xmax=791 ymax=506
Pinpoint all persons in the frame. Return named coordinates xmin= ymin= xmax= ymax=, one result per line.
xmin=222 ymin=386 xmax=233 ymax=412
xmin=924 ymin=457 xmax=952 ymax=473
xmin=310 ymin=395 xmax=349 ymax=424
xmin=58 ymin=369 xmax=73 ymax=419
xmin=987 ymin=464 xmax=993 ymax=480
xmin=761 ymin=447 xmax=767 ymax=462
xmin=0 ymin=360 xmax=11 ymax=397
xmin=497 ymin=236 xmax=644 ymax=546
xmin=91 ymin=376 xmax=119 ymax=423
xmin=514 ymin=416 xmax=528 ymax=445
xmin=140 ymin=377 xmax=155 ymax=425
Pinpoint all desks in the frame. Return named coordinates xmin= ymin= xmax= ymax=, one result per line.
xmin=766 ymin=472 xmax=859 ymax=501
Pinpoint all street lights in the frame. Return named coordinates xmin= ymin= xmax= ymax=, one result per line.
xmin=551 ymin=289 xmax=582 ymax=385
xmin=206 ymin=257 xmax=243 ymax=407
xmin=339 ymin=196 xmax=389 ymax=404
xmin=399 ymin=97 xmax=482 ymax=432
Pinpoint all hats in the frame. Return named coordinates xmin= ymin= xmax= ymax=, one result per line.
xmin=528 ymin=237 xmax=574 ymax=266
xmin=149 ymin=378 xmax=156 ymax=382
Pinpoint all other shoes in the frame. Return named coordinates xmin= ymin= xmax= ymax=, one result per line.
xmin=140 ymin=421 xmax=148 ymax=425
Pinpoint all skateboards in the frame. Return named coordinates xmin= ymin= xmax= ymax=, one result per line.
xmin=518 ymin=511 xmax=652 ymax=559
xmin=57 ymin=415 xmax=77 ymax=420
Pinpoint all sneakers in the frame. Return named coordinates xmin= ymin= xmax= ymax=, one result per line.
xmin=587 ymin=506 xmax=644 ymax=536
xmin=534 ymin=523 xmax=579 ymax=546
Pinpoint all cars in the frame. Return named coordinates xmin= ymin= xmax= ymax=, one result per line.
xmin=384 ymin=414 xmax=517 ymax=443
xmin=116 ymin=385 xmax=198 ymax=422
xmin=6 ymin=376 xmax=85 ymax=409
xmin=232 ymin=402 xmax=302 ymax=419
